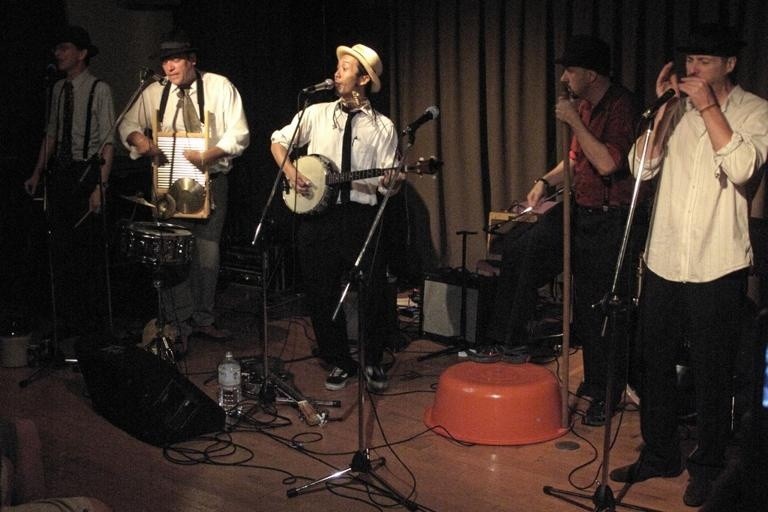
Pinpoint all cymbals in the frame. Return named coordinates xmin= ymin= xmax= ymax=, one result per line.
xmin=167 ymin=178 xmax=204 ymax=214
xmin=121 ymin=194 xmax=153 ymax=208
xmin=148 ymin=194 xmax=176 ymax=222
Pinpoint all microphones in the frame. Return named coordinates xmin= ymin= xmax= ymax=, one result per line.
xmin=302 ymin=78 xmax=334 ymax=94
xmin=144 ymin=66 xmax=168 ymax=85
xmin=399 ymin=105 xmax=440 ymax=138
xmin=642 ymin=88 xmax=676 ymax=120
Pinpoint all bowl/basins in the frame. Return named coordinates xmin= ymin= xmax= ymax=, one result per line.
xmin=423 ymin=363 xmax=573 ymax=446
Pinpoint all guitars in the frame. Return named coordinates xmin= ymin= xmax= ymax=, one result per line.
xmin=235 ymin=356 xmax=328 ymax=426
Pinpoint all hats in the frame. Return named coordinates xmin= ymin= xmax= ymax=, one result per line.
xmin=336 ymin=42 xmax=383 ymax=93
xmin=149 ymin=33 xmax=196 ymax=60
xmin=675 ymin=24 xmax=740 ymax=57
xmin=55 ymin=26 xmax=98 ymax=58
xmin=552 ymin=36 xmax=612 ymax=69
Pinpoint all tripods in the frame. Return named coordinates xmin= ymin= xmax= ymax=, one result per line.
xmin=286 ymin=269 xmax=421 ymax=511
xmin=19 ymin=173 xmax=81 ymax=390
xmin=227 ymin=223 xmax=342 ymax=432
xmin=543 ymin=296 xmax=657 ymax=512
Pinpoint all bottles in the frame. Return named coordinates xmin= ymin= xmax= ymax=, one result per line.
xmin=217 ymin=351 xmax=240 ymax=413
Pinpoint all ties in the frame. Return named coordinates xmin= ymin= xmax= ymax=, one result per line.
xmin=180 ymin=89 xmax=201 ymax=135
xmin=338 ymin=101 xmax=362 ymax=206
xmin=61 ymin=82 xmax=74 ymax=162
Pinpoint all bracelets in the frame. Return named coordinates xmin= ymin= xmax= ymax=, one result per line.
xmin=533 ymin=177 xmax=549 ymax=190
xmin=200 ymin=152 xmax=204 ymax=167
xmin=699 ymin=103 xmax=718 ymax=114
xmin=97 ymin=181 xmax=109 ymax=189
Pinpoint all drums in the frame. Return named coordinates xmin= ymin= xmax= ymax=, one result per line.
xmin=124 ymin=221 xmax=195 ymax=272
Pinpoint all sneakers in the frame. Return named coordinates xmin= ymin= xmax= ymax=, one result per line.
xmin=581 ymin=396 xmax=617 ymax=427
xmin=325 ymin=364 xmax=353 ymax=391
xmin=365 ymin=364 xmax=390 ymax=392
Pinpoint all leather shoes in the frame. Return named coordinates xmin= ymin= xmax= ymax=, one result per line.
xmin=193 ymin=321 xmax=228 ymax=340
xmin=683 ymin=476 xmax=714 ymax=507
xmin=610 ymin=458 xmax=683 ymax=483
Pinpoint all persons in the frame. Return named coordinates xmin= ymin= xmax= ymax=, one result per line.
xmin=114 ymin=30 xmax=252 ymax=362
xmin=609 ymin=21 xmax=768 ymax=507
xmin=467 ymin=42 xmax=653 ymax=426
xmin=270 ymin=43 xmax=406 ymax=392
xmin=24 ymin=24 xmax=116 ymax=214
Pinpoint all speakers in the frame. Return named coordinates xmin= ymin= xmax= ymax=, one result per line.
xmin=81 ymin=343 xmax=226 ymax=447
